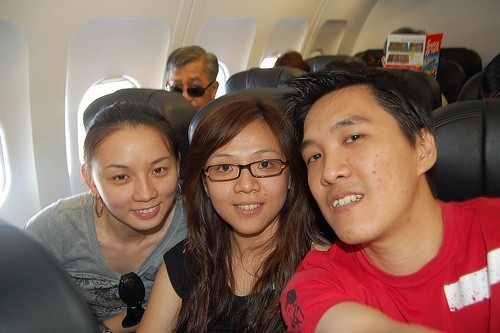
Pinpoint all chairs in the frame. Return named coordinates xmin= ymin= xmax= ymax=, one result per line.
xmin=225 ymin=45 xmax=500 ymax=199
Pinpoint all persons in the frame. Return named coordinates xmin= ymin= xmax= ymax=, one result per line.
xmin=278 ymin=66 xmax=500 ymax=333
xmin=134 ymin=98 xmax=332 ymax=333
xmin=158 ymin=27 xmax=500 ymax=102
xmin=21 ymin=100 xmax=189 ymax=333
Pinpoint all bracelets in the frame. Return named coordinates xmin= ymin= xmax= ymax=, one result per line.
xmin=98 ymin=322 xmax=112 ymax=333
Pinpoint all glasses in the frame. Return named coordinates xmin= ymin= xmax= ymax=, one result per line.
xmin=118 ymin=272 xmax=147 ymax=328
xmin=203 ymin=159 xmax=289 ymax=182
xmin=164 ymin=81 xmax=216 ymax=97
